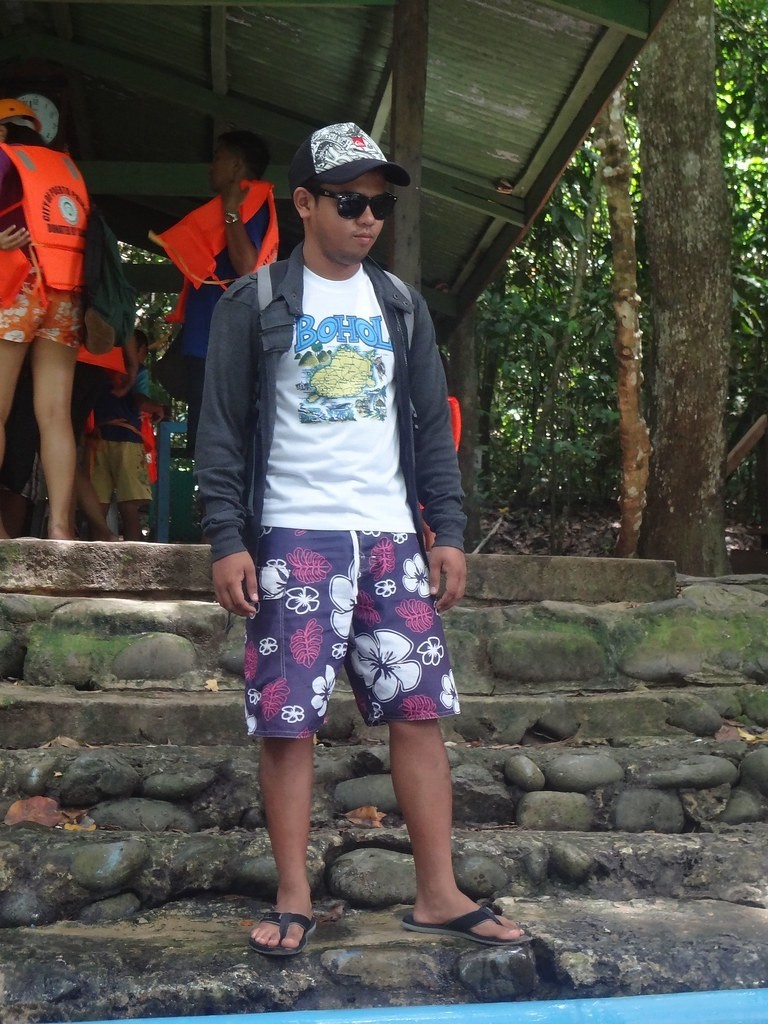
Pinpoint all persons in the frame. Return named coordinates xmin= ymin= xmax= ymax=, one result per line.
xmin=1 ymin=92 xmax=280 ymax=542
xmin=195 ymin=122 xmax=533 ymax=956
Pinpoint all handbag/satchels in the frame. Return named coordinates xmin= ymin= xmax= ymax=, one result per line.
xmin=81 ymin=209 xmax=136 ymax=346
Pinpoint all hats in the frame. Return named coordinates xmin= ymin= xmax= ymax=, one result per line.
xmin=287 ymin=122 xmax=411 ymax=199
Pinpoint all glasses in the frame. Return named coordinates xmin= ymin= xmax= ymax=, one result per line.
xmin=307 ymin=187 xmax=398 ymax=221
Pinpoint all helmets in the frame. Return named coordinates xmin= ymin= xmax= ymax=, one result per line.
xmin=0 ymin=98 xmax=40 ymax=134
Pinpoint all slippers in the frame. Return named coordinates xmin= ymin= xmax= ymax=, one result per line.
xmin=401 ymin=906 xmax=531 ymax=947
xmin=250 ymin=911 xmax=316 ymax=956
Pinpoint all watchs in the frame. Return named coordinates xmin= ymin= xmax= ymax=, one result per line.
xmin=225 ymin=211 xmax=239 ymax=224
